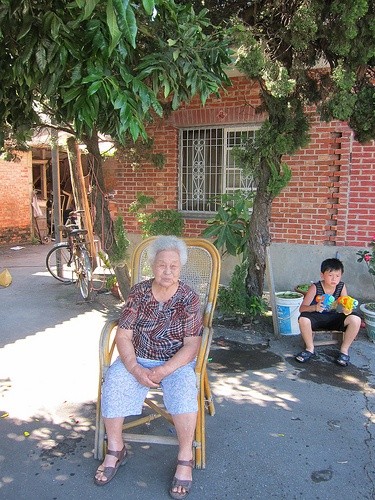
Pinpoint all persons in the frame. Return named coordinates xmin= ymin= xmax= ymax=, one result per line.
xmin=295 ymin=258 xmax=362 ymax=367
xmin=94 ymin=236 xmax=202 ymax=499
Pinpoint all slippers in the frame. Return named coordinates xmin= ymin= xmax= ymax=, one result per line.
xmin=336 ymin=352 xmax=349 ymax=366
xmin=296 ymin=350 xmax=315 ymax=363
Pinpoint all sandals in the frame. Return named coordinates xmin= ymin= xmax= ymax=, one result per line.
xmin=95 ymin=444 xmax=128 ymax=485
xmin=169 ymin=459 xmax=195 ymax=499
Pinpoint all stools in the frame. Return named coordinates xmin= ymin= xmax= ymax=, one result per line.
xmin=298 ymin=331 xmax=345 ymax=351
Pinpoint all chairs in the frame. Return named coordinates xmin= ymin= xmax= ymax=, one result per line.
xmin=94 ymin=237 xmax=223 ymax=470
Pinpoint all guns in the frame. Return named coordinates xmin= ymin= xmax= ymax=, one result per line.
xmin=336 ymin=295 xmax=358 ymax=313
xmin=316 ymin=293 xmax=335 ymax=313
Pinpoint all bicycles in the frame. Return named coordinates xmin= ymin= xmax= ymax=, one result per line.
xmin=46 ymin=210 xmax=95 ymax=302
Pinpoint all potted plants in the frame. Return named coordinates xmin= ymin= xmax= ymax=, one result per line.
xmin=295 ymin=284 xmax=311 ymax=298
xmin=98 ymin=216 xmax=131 ymax=300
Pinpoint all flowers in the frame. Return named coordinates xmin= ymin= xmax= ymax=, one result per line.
xmin=355 ymin=233 xmax=375 ymax=289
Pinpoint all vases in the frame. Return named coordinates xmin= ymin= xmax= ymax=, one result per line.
xmin=360 ymin=302 xmax=375 ymax=343
